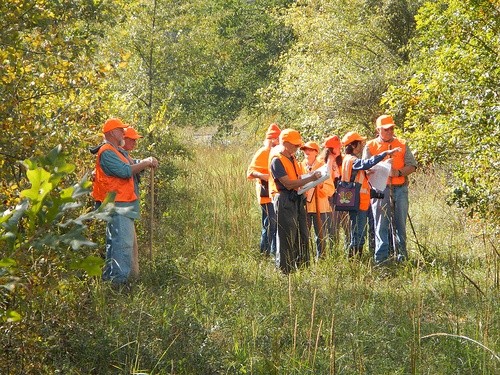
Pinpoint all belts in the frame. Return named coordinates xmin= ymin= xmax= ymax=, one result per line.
xmin=386 ymin=182 xmax=405 ymax=187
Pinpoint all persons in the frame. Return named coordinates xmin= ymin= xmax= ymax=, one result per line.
xmin=89 ymin=118 xmax=159 ymax=288
xmin=122 ymin=128 xmax=143 ymax=276
xmin=268 ymin=129 xmax=321 ymax=275
xmin=300 ymin=141 xmax=336 ymax=260
xmin=342 ymin=131 xmax=402 ymax=258
xmin=247 ymin=123 xmax=281 ymax=252
xmin=324 ymin=135 xmax=349 ymax=255
xmin=362 ymin=116 xmax=417 ymax=261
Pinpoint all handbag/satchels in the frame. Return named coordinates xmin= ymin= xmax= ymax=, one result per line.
xmin=335 ymin=179 xmax=363 ymax=212
xmin=260 ymin=179 xmax=269 ymax=198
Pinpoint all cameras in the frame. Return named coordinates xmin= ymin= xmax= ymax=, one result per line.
xmin=370 ymin=188 xmax=384 ymax=199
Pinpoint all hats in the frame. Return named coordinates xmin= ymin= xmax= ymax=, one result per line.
xmin=324 ymin=135 xmax=342 ymax=148
xmin=342 ymin=131 xmax=367 ymax=145
xmin=103 ymin=119 xmax=129 ymax=134
xmin=279 ymin=128 xmax=303 ymax=146
xmin=300 ymin=141 xmax=319 ymax=152
xmin=123 ymin=127 xmax=144 ymax=139
xmin=376 ymin=114 xmax=396 ymax=129
xmin=266 ymin=124 xmax=281 ymax=139
